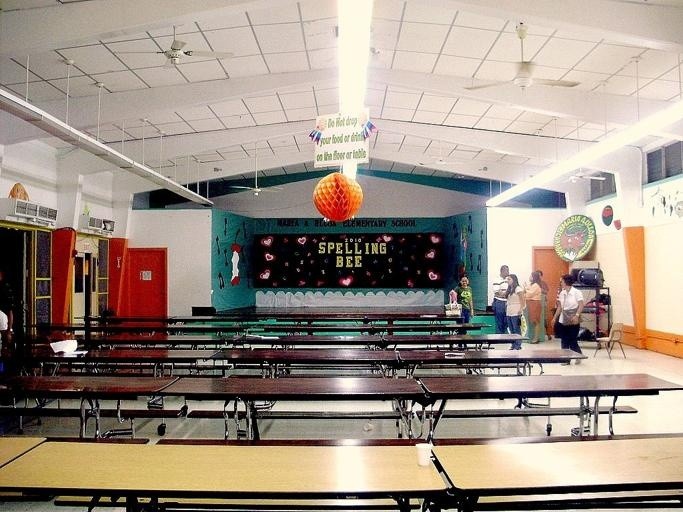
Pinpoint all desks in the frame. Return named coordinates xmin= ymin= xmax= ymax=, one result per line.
xmin=0 ymin=313 xmax=682 ymax=512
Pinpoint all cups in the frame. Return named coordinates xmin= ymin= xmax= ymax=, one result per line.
xmin=415 ymin=444 xmax=433 ymax=467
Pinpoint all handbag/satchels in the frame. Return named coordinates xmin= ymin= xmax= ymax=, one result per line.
xmin=445 ymin=303 xmax=462 ymax=317
xmin=562 ymin=308 xmax=583 ymax=325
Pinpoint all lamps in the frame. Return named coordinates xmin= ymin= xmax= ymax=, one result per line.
xmin=0 ymin=52 xmax=213 ymax=207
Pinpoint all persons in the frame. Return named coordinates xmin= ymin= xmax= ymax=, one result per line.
xmin=550 ymin=275 xmax=587 ymax=366
xmin=454 ymin=274 xmax=474 ymax=348
xmin=488 ymin=262 xmax=551 ymax=350
xmin=554 ymin=276 xmax=565 ymax=308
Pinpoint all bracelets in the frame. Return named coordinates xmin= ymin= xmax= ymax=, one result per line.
xmin=575 ymin=313 xmax=580 ymax=317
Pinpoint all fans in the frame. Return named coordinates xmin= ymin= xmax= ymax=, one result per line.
xmin=115 ymin=25 xmax=234 ymax=71
xmin=460 ymin=22 xmax=581 ymax=93
